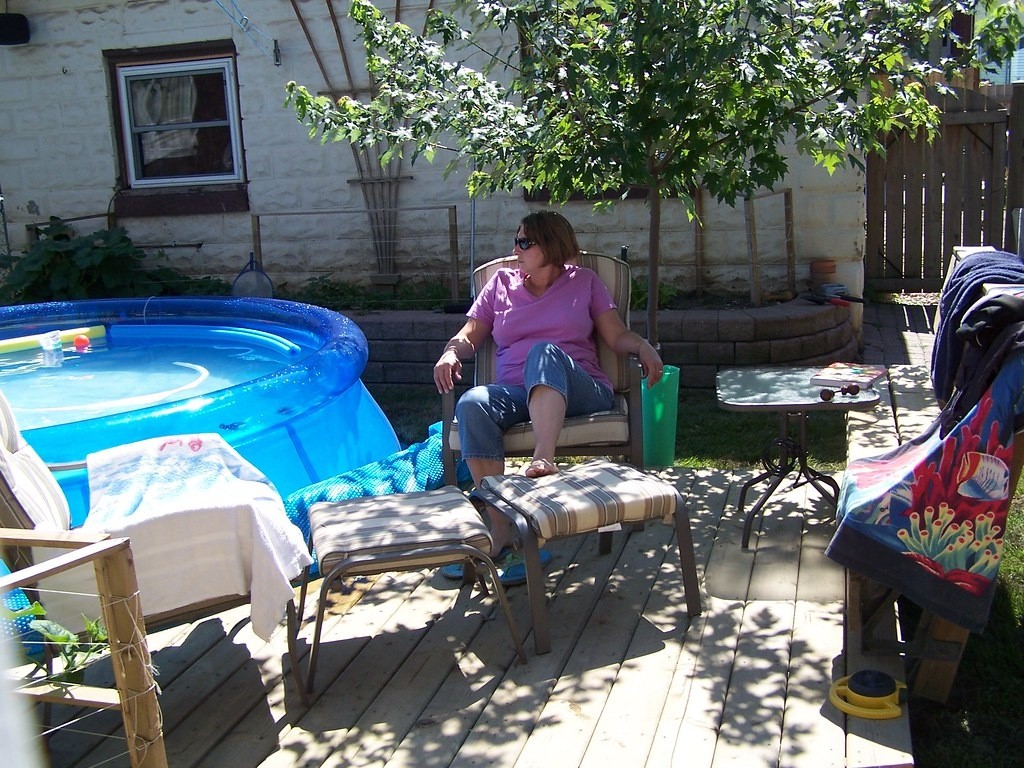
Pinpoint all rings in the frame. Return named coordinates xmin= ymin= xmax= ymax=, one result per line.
xmin=659 ymin=371 xmax=663 ymax=372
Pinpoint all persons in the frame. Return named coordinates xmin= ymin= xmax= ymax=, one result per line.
xmin=434 ymin=212 xmax=664 ymax=558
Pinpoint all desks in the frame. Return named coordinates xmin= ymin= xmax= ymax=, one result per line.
xmin=715 ymin=367 xmax=880 ymax=547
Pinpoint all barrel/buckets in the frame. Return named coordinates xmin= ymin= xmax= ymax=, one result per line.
xmin=642 ymin=365 xmax=681 ymax=467
xmin=810 ymin=260 xmax=836 ymax=293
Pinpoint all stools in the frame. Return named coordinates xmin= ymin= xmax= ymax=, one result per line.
xmin=293 ymin=485 xmax=529 ymax=694
xmin=479 ymin=460 xmax=702 ymax=655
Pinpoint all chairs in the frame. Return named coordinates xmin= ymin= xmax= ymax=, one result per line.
xmin=0 ymin=395 xmax=304 ymax=699
xmin=439 ymin=251 xmax=645 ymax=554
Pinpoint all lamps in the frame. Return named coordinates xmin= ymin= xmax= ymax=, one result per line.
xmin=0 ymin=0 xmax=31 ymax=47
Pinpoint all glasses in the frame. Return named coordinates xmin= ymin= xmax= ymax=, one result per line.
xmin=515 ymin=237 xmax=536 ymax=250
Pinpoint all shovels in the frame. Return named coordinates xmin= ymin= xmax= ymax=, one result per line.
xmin=800 ymin=294 xmax=850 ymax=307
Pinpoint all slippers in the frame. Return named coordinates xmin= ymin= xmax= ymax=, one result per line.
xmin=442 ymin=547 xmax=507 ymax=578
xmin=499 ymin=548 xmax=552 ymax=585
